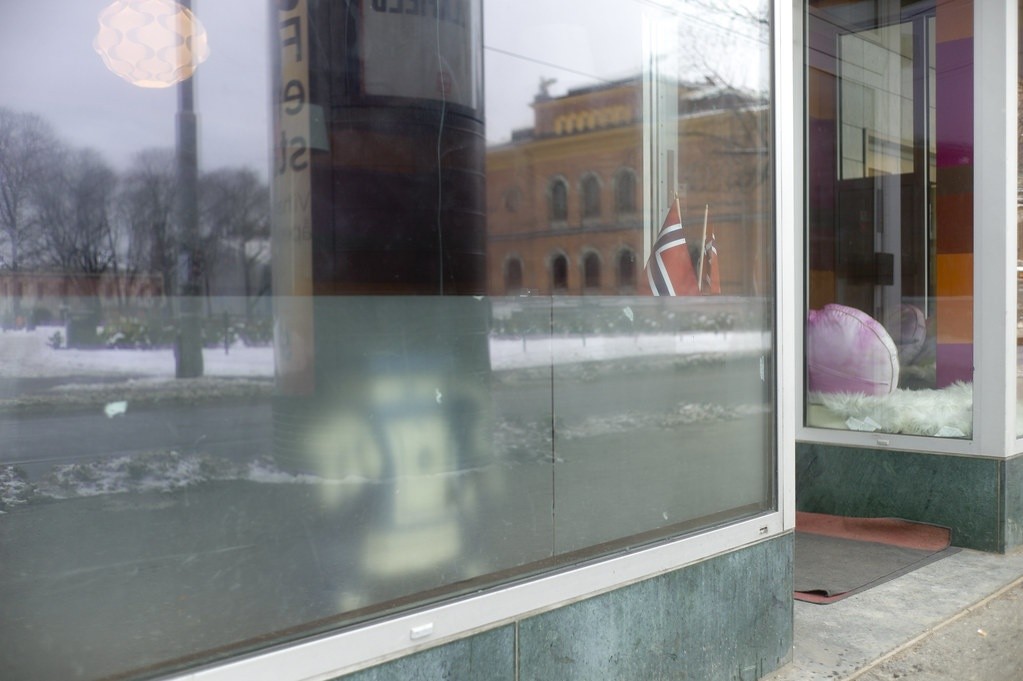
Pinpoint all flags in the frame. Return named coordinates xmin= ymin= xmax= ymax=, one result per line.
xmin=699 ymin=206 xmax=721 ymax=294
xmin=635 ymin=198 xmax=699 ymax=295
